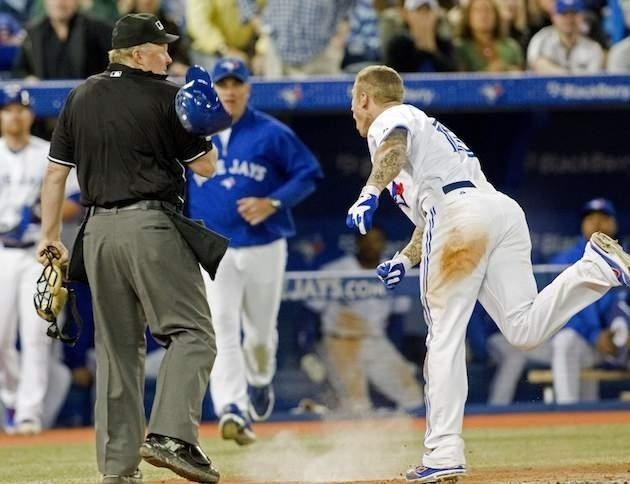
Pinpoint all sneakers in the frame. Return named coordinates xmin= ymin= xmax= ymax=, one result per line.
xmin=217 ymin=401 xmax=260 ymax=446
xmin=137 ymin=430 xmax=221 ymax=484
xmin=587 ymin=229 xmax=630 ymax=293
xmin=402 ymin=459 xmax=470 ymax=483
xmin=251 ymin=386 xmax=278 ymax=423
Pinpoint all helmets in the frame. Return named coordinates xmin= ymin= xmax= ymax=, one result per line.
xmin=169 ymin=63 xmax=235 ymax=141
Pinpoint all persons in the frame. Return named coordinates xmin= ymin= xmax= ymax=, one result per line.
xmin=345 ymin=64 xmax=629 ymax=482
xmin=292 ymin=196 xmax=629 ymax=410
xmin=0 ymin=1 xmax=628 ymax=82
xmin=1 ymin=11 xmax=326 ymax=484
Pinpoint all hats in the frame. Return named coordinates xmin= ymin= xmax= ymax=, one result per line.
xmin=556 ymin=0 xmax=584 ymax=13
xmin=579 ymin=195 xmax=618 ymax=219
xmin=108 ymin=12 xmax=183 ymax=48
xmin=0 ymin=84 xmax=36 ymax=110
xmin=209 ymin=56 xmax=254 ymax=83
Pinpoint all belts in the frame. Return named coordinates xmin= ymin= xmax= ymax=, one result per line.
xmin=93 ymin=200 xmax=162 ymax=215
xmin=442 ymin=180 xmax=477 ymax=194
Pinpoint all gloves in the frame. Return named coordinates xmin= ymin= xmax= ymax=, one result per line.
xmin=374 ymin=249 xmax=413 ymax=291
xmin=343 ymin=185 xmax=380 ymax=235
